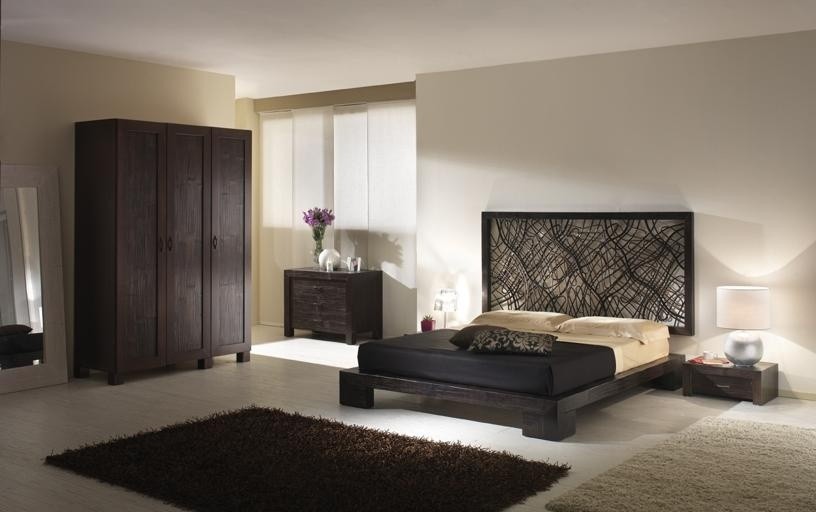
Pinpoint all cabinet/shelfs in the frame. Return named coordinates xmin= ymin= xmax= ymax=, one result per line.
xmin=72 ymin=118 xmax=252 ymax=386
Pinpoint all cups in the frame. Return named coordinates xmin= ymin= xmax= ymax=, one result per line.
xmin=327 ymin=257 xmax=333 ymax=273
xmin=703 ymin=352 xmax=714 ymax=361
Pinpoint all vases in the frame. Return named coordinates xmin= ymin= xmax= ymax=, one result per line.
xmin=311 ymin=239 xmax=323 ymax=266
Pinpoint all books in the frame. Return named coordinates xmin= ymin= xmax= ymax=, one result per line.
xmin=702 ymin=359 xmax=729 ymax=365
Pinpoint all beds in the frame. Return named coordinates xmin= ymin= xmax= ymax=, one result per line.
xmin=338 ymin=327 xmax=686 ymax=441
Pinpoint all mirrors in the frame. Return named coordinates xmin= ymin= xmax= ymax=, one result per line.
xmin=0 ymin=187 xmax=45 ymax=372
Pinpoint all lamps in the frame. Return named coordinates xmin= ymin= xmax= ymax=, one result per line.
xmin=715 ymin=286 xmax=771 ymax=367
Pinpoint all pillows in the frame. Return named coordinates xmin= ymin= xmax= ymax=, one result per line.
xmin=468 ymin=329 xmax=558 ymax=356
xmin=554 ymin=316 xmax=670 ymax=345
xmin=449 ymin=325 xmax=509 ymax=350
xmin=1 ymin=324 xmax=31 ymax=337
xmin=468 ymin=309 xmax=576 ymax=332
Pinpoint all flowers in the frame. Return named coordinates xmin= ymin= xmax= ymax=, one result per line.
xmin=301 ymin=207 xmax=335 ymax=256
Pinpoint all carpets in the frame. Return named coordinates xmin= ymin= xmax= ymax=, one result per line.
xmin=544 ymin=411 xmax=816 ymax=512
xmin=716 ymin=395 xmax=816 ymax=432
xmin=40 ymin=405 xmax=573 ymax=512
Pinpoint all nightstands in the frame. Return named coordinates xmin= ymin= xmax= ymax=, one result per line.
xmin=283 ymin=267 xmax=383 ymax=345
xmin=683 ymin=355 xmax=778 ymax=406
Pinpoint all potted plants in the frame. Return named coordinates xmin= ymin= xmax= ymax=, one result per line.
xmin=420 ymin=314 xmax=436 ymax=332
